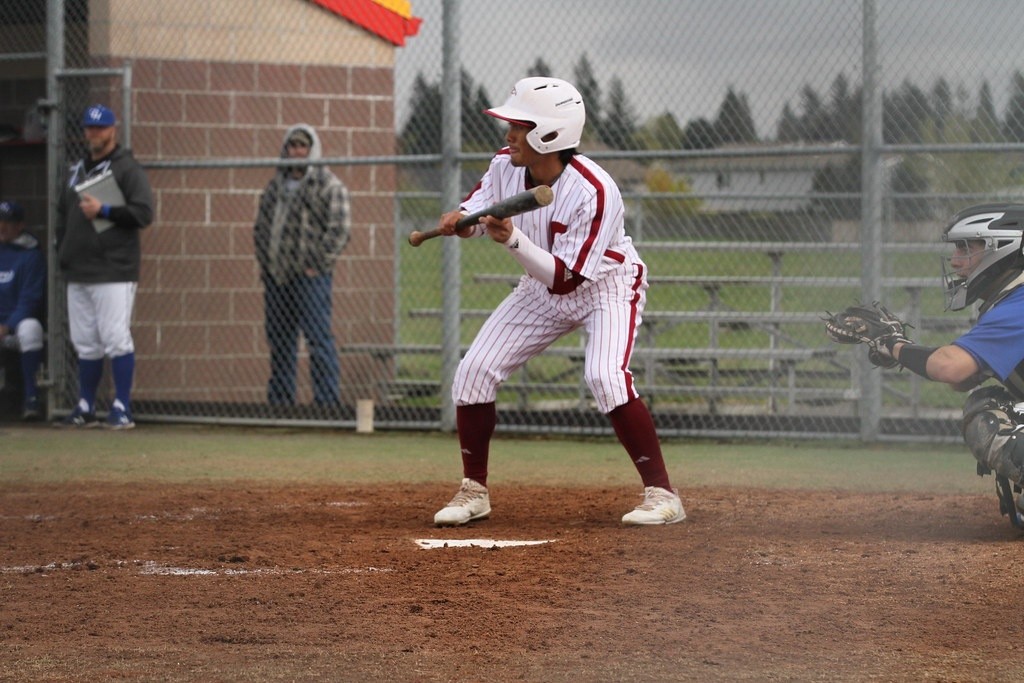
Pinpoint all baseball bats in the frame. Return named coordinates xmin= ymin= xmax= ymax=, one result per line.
xmin=408 ymin=184 xmax=553 ymax=248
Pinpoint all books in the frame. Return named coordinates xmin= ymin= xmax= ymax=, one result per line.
xmin=74 ymin=170 xmax=126 ymax=234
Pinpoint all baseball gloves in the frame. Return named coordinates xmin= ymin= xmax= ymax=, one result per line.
xmin=824 ymin=299 xmax=905 ymax=369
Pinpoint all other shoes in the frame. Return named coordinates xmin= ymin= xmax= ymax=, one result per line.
xmin=21 ymin=398 xmax=43 ymax=422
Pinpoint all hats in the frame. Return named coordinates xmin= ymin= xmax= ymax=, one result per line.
xmin=0 ymin=200 xmax=23 ymax=221
xmin=80 ymin=103 xmax=116 ymax=127
xmin=290 ymin=129 xmax=313 ymax=148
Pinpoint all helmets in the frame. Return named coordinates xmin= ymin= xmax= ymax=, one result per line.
xmin=940 ymin=202 xmax=1024 ymax=312
xmin=484 ymin=76 xmax=586 ymax=154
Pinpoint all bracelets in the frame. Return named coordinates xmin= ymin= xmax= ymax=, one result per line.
xmin=898 ymin=344 xmax=941 ymax=382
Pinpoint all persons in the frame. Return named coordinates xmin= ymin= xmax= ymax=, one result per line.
xmin=51 ymin=105 xmax=154 ymax=429
xmin=252 ymin=122 xmax=353 ymax=409
xmin=0 ymin=200 xmax=47 ymax=421
xmin=434 ymin=76 xmax=687 ymax=525
xmin=828 ymin=203 xmax=1024 ymax=534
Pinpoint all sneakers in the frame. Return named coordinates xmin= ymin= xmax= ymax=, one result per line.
xmin=101 ymin=406 xmax=136 ymax=430
xmin=53 ymin=404 xmax=100 ymax=429
xmin=621 ymin=486 xmax=687 ymax=525
xmin=433 ymin=478 xmax=491 ymax=525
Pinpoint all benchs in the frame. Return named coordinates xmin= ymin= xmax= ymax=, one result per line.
xmin=336 ymin=239 xmax=956 ymax=400
